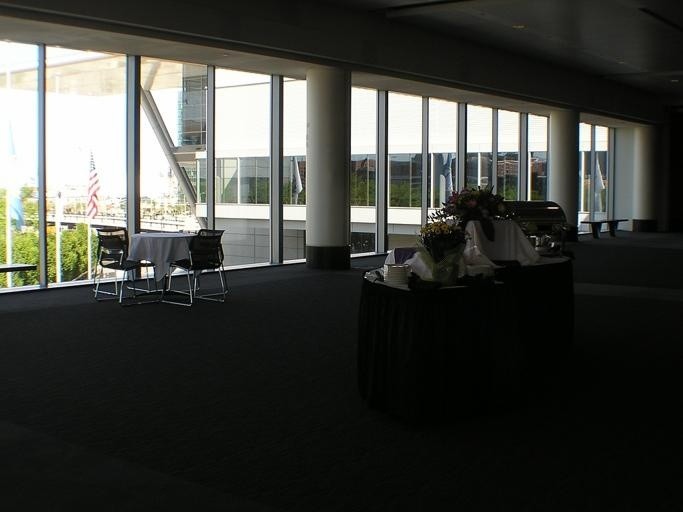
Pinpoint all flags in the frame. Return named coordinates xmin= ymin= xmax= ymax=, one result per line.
xmin=85 ymin=151 xmax=102 ymax=221
xmin=6 ymin=120 xmax=26 ymax=233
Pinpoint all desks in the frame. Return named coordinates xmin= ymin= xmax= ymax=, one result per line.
xmin=358 ymin=252 xmax=577 ymax=420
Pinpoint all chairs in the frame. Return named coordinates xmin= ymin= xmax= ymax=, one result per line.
xmin=91 ymin=227 xmax=231 ymax=308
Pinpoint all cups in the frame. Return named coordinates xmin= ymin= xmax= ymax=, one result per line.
xmin=384 ymin=264 xmax=409 ymax=285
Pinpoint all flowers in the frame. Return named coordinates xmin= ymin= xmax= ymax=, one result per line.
xmin=416 ymin=184 xmax=504 ymax=251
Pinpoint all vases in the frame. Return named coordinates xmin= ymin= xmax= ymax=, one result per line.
xmin=432 ymin=242 xmax=447 ymax=280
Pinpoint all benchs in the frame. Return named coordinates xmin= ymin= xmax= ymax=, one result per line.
xmin=580 ymin=218 xmax=630 ymax=239
